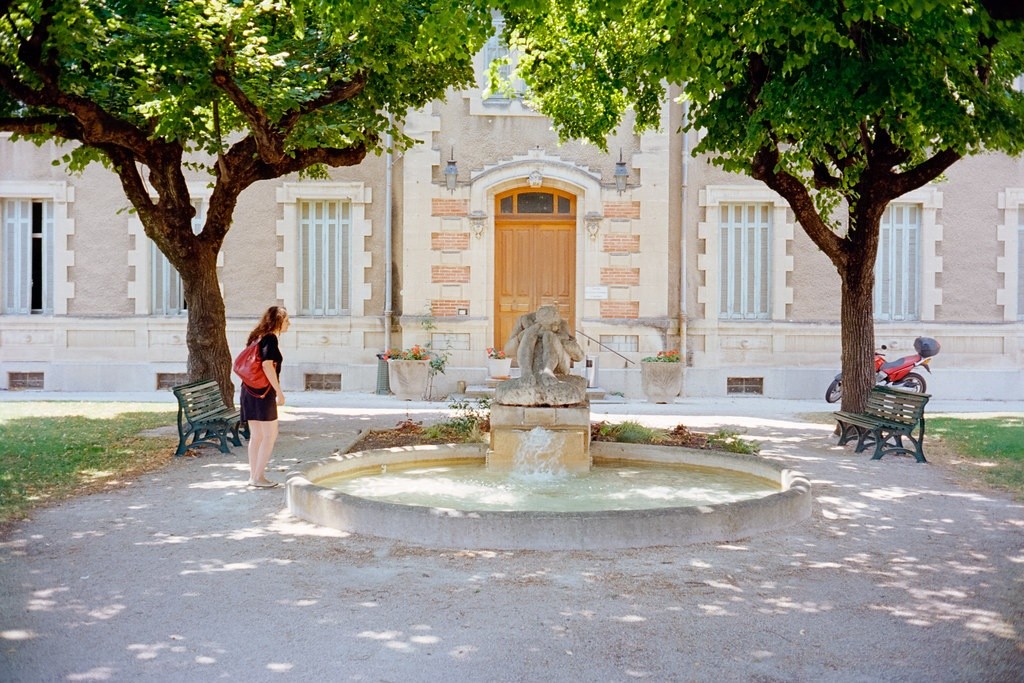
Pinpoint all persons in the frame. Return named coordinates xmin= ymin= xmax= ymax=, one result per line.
xmin=240 ymin=306 xmax=291 ymax=487
xmin=505 ymin=306 xmax=584 ymax=385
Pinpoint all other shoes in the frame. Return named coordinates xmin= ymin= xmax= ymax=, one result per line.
xmin=248 ymin=478 xmax=278 ymax=487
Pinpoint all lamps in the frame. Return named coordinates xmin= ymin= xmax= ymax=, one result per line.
xmin=445 ymin=146 xmax=458 ymax=195
xmin=612 ymin=147 xmax=628 ymax=196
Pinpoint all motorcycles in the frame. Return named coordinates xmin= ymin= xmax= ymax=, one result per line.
xmin=826 ymin=335 xmax=942 ymax=404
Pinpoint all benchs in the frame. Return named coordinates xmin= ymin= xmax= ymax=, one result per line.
xmin=833 ymin=385 xmax=932 ymax=463
xmin=172 ymin=378 xmax=250 ymax=456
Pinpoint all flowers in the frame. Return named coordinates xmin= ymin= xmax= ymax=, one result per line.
xmin=487 ymin=347 xmax=507 ymax=359
xmin=383 ymin=344 xmax=449 ymax=376
xmin=641 ymin=351 xmax=681 ymax=363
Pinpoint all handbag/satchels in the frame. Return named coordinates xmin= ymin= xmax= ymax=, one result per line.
xmin=234 ymin=332 xmax=277 ymax=399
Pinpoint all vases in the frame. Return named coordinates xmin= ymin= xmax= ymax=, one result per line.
xmin=641 ymin=363 xmax=683 ymax=404
xmin=387 ymin=359 xmax=431 ymax=401
xmin=488 ymin=357 xmax=511 ymax=378
